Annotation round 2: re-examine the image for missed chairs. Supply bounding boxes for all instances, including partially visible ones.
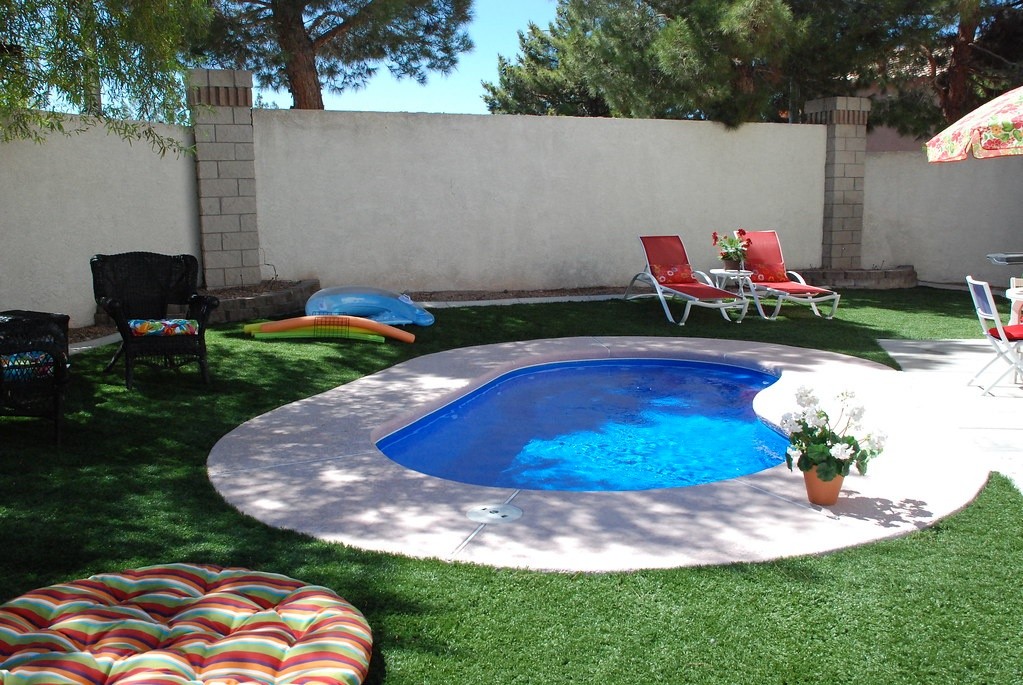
[88,251,219,392]
[732,229,841,320]
[1007,276,1023,384]
[965,273,1023,395]
[0,310,70,449]
[623,234,749,326]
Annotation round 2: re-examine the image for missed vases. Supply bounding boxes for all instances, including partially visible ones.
[803,464,845,506]
[723,259,740,272]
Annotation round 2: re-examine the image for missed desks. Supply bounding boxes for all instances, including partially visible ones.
[708,268,753,312]
[1004,286,1022,302]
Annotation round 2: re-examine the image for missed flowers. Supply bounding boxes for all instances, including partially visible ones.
[778,385,888,481]
[710,227,752,261]
